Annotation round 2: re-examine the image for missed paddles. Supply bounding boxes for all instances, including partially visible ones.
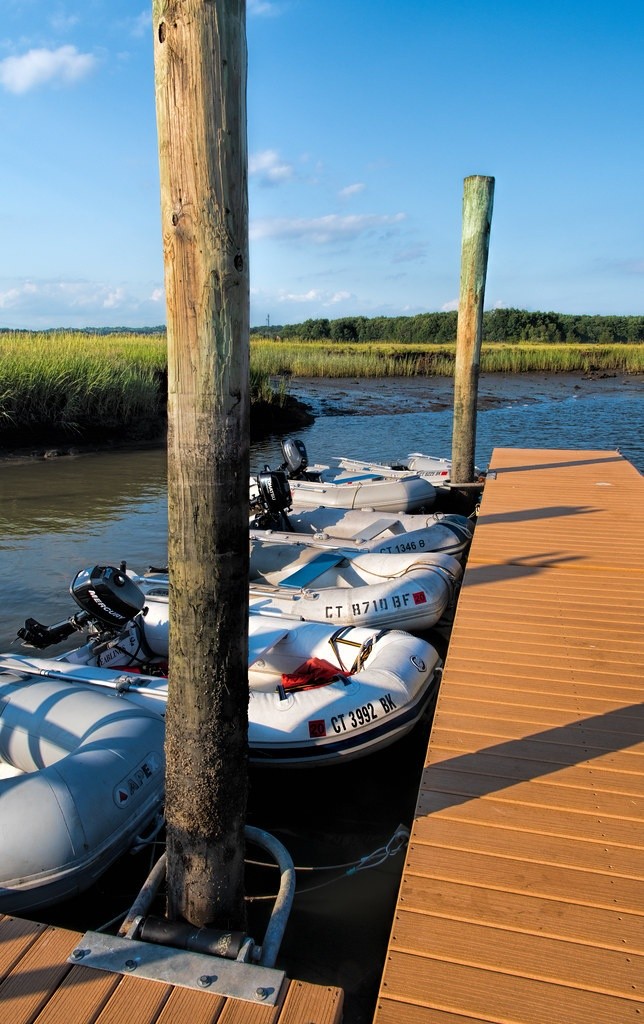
[250,476,325,493]
[0,656,168,697]
[143,593,303,622]
[118,568,295,600]
[406,452,478,470]
[331,456,391,470]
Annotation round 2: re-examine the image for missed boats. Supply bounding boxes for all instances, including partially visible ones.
[247,464,476,563]
[0,665,168,914]
[269,436,437,514]
[6,563,442,773]
[114,534,464,633]
[331,450,486,496]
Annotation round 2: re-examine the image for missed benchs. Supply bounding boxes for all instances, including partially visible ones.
[352,519,398,541]
[249,626,289,669]
[326,474,381,484]
[278,552,344,588]
